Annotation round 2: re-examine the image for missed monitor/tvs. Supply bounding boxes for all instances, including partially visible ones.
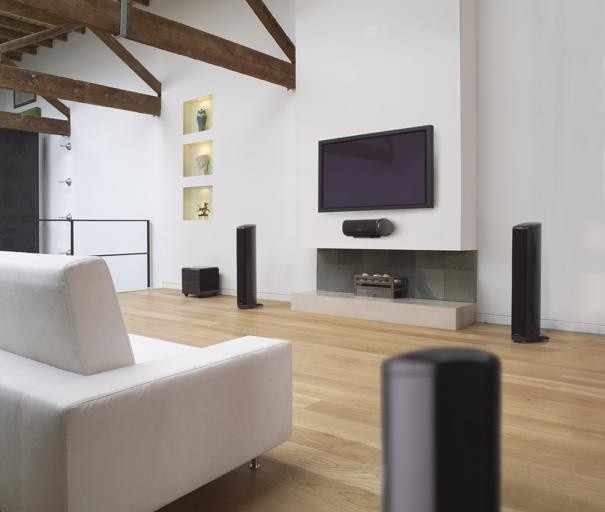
[317,125,436,211]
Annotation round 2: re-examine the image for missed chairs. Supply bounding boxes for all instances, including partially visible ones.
[2,249,294,512]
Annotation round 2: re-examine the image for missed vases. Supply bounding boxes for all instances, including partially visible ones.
[196,111,206,132]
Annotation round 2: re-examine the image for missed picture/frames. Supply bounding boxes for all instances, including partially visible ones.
[13,88,36,108]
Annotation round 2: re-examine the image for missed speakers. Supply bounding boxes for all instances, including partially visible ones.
[511,222,548,343]
[341,219,395,238]
[236,224,263,308]
